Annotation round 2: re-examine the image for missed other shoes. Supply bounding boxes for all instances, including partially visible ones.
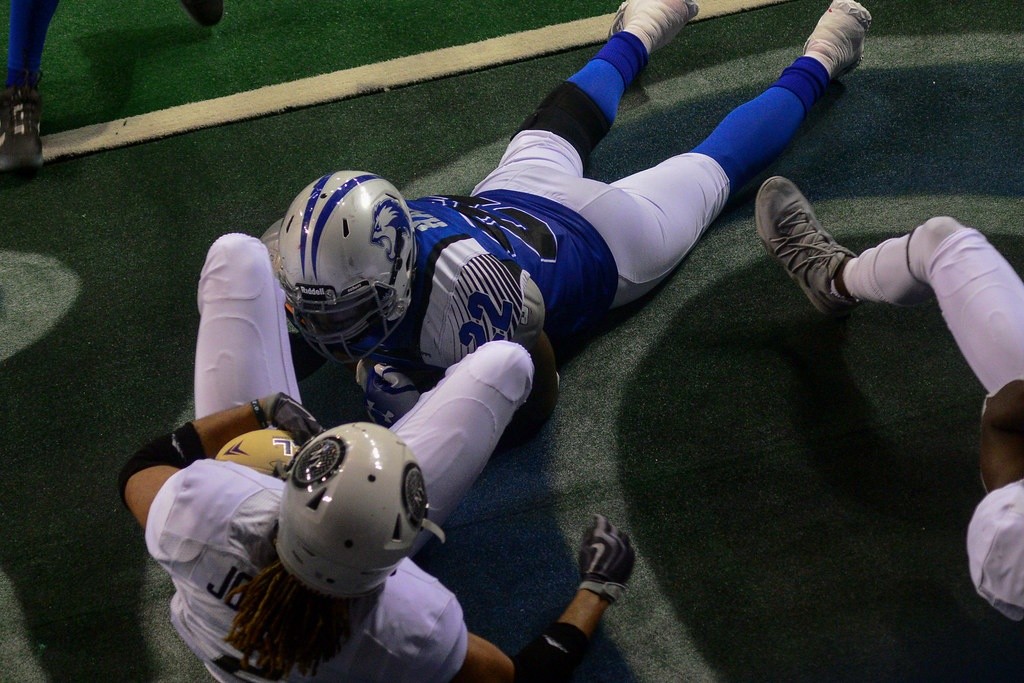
[607,0,699,40]
[0,70,44,171]
[804,0,872,74]
[181,0,224,25]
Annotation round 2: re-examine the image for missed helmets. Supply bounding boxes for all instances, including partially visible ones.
[278,170,417,362]
[275,422,430,596]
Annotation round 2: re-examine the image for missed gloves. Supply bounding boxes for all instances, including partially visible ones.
[266,391,324,447]
[364,362,420,429]
[579,514,635,604]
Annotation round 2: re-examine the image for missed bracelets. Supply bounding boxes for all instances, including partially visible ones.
[251,399,268,429]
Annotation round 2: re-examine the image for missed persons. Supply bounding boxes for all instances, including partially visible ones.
[118,231,637,683]
[0,0,223,174]
[256,0,874,427]
[755,176,1024,623]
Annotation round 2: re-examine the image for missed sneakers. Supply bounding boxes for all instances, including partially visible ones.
[754,176,863,319]
